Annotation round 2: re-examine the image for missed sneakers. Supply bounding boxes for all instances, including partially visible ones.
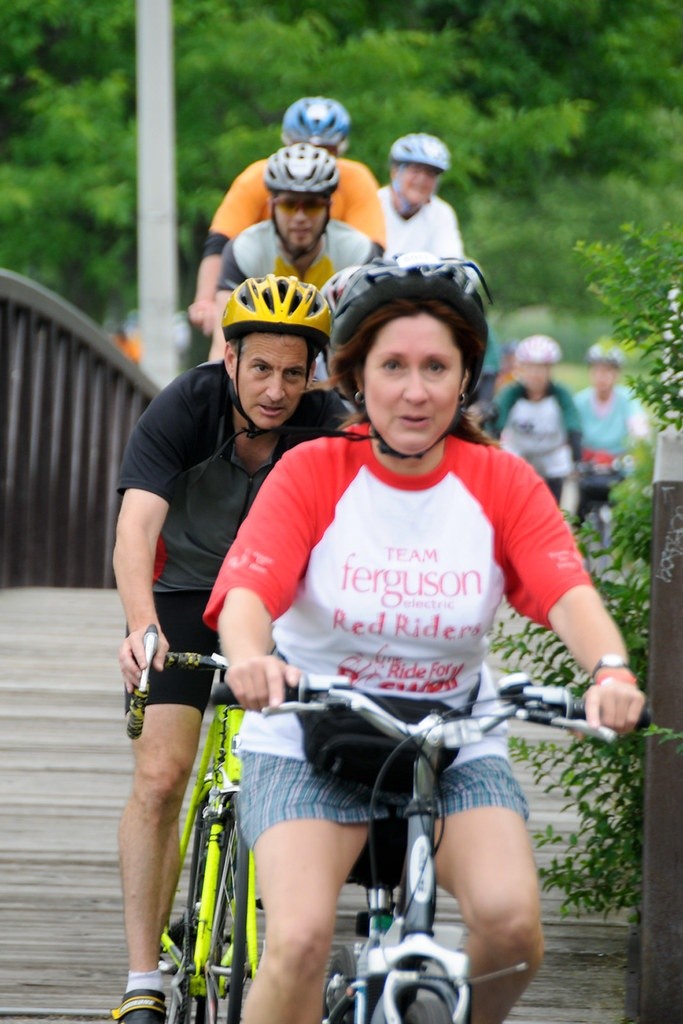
[110,988,169,1024]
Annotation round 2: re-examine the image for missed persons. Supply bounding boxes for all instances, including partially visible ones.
[185,98,385,335]
[486,336,584,516]
[112,276,356,1024]
[570,340,647,528]
[211,144,390,385]
[374,132,468,271]
[204,256,646,1024]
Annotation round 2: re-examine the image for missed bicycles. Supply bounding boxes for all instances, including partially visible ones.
[575,457,638,551]
[210,672,654,1021]
[128,627,268,1024]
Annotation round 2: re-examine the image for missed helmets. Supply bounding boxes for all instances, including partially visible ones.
[261,142,341,200]
[514,334,564,366]
[220,274,333,361]
[388,131,452,175]
[319,250,496,400]
[582,341,627,370]
[280,95,352,148]
[499,338,521,355]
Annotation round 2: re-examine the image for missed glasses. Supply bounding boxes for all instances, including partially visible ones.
[273,197,328,218]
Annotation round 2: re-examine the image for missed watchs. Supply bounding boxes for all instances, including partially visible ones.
[591,654,633,677]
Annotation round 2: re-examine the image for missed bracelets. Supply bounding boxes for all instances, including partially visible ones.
[596,671,638,686]
